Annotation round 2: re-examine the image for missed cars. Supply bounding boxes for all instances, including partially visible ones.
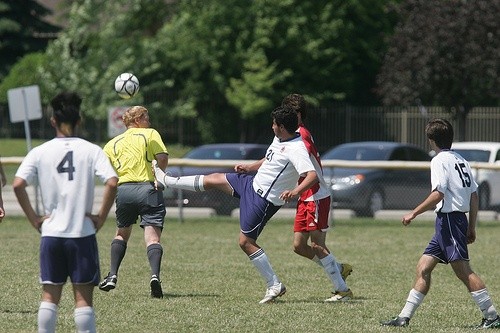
[163,143,269,216]
[320,141,432,217]
[451,141,500,210]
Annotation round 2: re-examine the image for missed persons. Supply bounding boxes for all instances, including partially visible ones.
[282,94,354,303]
[99,106,169,299]
[380,118,500,329]
[151,107,319,303]
[0,160,6,223]
[13,92,120,332]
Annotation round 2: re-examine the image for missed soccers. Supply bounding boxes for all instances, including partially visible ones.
[115,72,139,99]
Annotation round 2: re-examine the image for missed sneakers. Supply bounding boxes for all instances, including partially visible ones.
[151,160,168,192]
[149,275,163,299]
[473,315,500,330]
[335,264,353,295]
[259,282,286,304]
[379,316,410,328]
[325,289,353,303]
[99,272,117,292]
